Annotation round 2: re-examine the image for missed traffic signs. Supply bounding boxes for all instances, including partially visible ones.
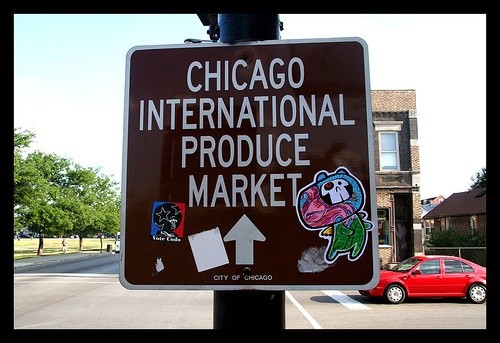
[120,35,380,292]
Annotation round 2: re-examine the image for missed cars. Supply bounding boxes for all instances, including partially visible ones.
[359,255,486,304]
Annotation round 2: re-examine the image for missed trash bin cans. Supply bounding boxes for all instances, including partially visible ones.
[107,244,112,252]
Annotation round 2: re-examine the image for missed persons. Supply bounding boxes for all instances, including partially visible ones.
[62,238,67,255]
